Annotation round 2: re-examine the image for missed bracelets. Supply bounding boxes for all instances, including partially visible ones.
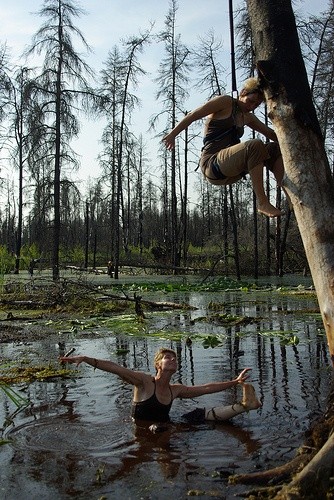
[93,358,98,374]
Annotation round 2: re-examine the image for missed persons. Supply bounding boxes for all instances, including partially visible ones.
[56,346,261,434]
[161,76,294,218]
